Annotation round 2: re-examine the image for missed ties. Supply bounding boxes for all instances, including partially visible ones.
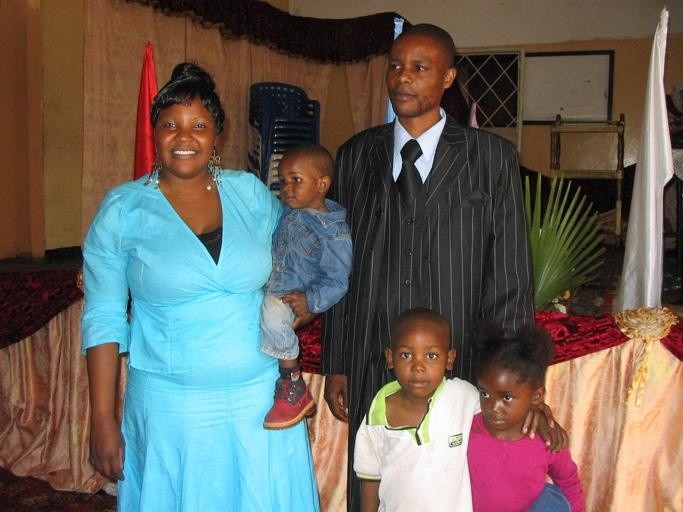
[395,139,423,210]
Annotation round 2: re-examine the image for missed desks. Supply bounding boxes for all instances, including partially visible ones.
[0,244,683,512]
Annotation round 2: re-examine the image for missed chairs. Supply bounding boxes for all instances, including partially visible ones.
[246,81,321,200]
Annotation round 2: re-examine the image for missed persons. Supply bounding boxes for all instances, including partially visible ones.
[322,23,553,512]
[352,307,570,512]
[466,316,586,511]
[79,63,320,512]
[258,144,353,430]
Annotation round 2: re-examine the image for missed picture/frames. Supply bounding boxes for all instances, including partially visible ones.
[515,50,614,125]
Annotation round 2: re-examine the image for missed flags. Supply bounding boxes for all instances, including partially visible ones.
[613,5,673,313]
[132,41,160,180]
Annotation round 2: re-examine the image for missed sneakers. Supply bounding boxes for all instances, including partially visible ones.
[263,375,317,429]
